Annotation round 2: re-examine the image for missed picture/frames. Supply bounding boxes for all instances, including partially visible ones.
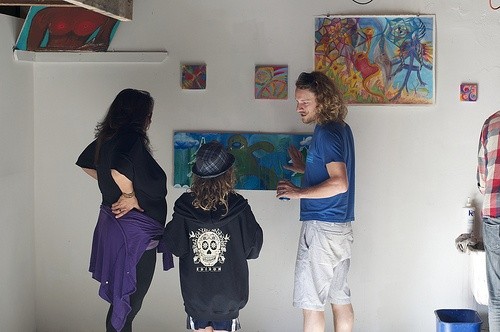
[311,12,438,108]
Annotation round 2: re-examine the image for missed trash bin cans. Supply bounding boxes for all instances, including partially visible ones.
[434,308,484,332]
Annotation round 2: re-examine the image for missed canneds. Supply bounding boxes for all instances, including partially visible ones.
[279,178,290,201]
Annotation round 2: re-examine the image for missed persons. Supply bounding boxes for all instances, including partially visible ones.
[74,89,168,332]
[275,71,355,332]
[163,143,264,332]
[476,110,500,332]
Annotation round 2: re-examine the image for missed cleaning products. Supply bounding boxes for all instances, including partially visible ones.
[461,197,476,234]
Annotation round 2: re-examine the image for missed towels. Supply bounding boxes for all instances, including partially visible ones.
[455,234,477,255]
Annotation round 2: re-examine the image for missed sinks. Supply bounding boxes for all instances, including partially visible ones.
[467,244,489,305]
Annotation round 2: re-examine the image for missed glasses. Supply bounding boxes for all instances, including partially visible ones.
[299,72,321,86]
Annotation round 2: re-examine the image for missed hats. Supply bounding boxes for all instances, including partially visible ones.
[192,142,235,179]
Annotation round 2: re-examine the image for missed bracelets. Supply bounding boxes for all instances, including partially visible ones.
[122,192,135,198]
